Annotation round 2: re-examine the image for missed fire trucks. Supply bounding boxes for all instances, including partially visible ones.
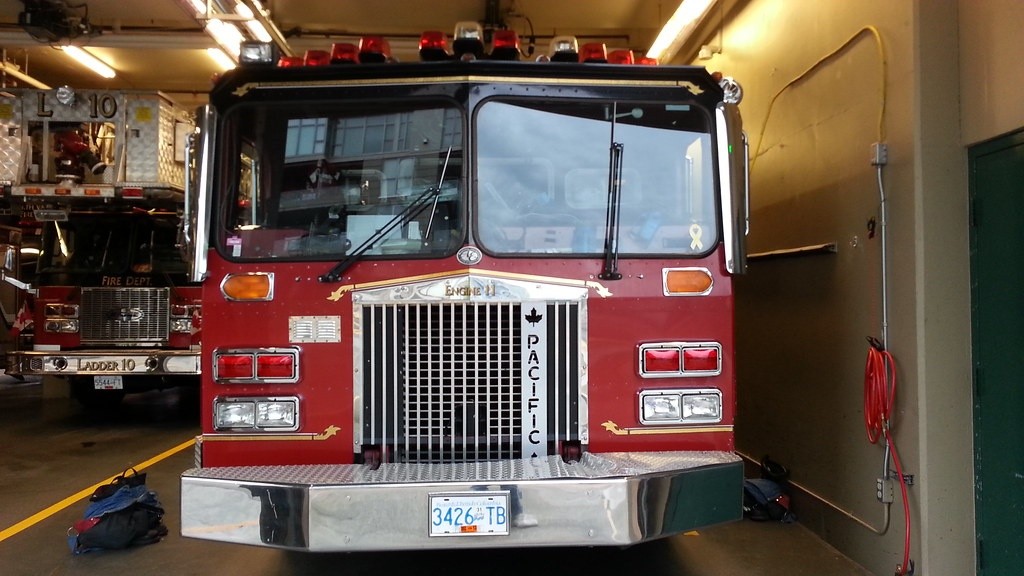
[179,24,748,553]
[1,82,251,403]
